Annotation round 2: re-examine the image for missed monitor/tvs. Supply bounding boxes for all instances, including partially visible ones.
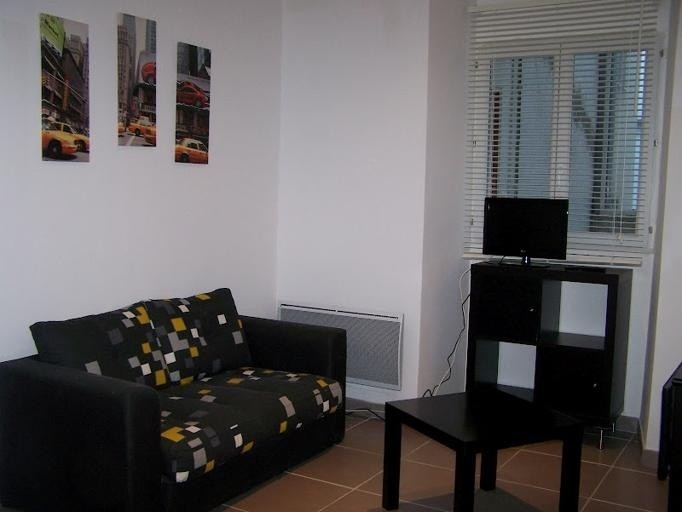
[482,197,569,269]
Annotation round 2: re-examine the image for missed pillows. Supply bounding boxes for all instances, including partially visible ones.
[29,299,171,390]
[149,287,253,386]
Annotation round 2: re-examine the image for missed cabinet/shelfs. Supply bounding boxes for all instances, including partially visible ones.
[658,360,682,512]
[465,261,633,449]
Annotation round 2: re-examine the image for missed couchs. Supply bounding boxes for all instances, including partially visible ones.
[0,315,347,512]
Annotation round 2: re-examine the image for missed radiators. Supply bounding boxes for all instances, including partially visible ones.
[278,302,403,391]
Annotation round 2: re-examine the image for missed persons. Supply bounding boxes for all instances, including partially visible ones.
[47,109,58,122]
